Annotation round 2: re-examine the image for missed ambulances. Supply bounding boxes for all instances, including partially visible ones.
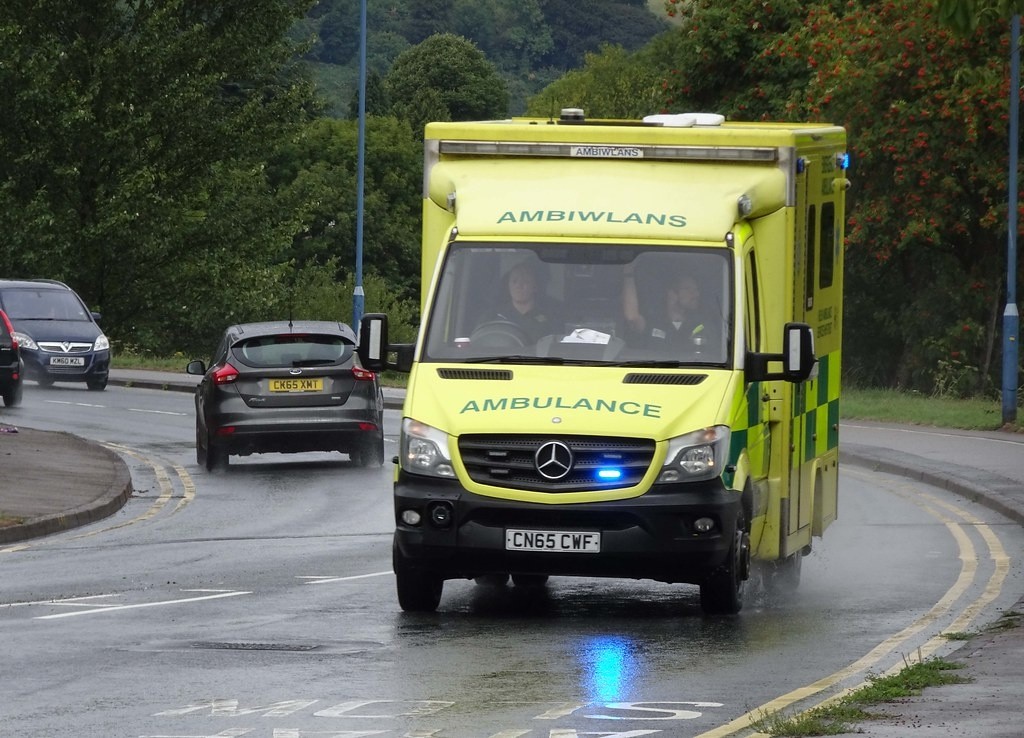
[359,107,851,613]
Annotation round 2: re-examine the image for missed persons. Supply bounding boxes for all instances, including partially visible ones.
[471,266,559,346]
[622,252,719,352]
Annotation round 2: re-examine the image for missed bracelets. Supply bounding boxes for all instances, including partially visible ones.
[624,273,634,277]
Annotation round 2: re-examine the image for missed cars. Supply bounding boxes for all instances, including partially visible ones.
[186,320,384,470]
[0,279,110,391]
